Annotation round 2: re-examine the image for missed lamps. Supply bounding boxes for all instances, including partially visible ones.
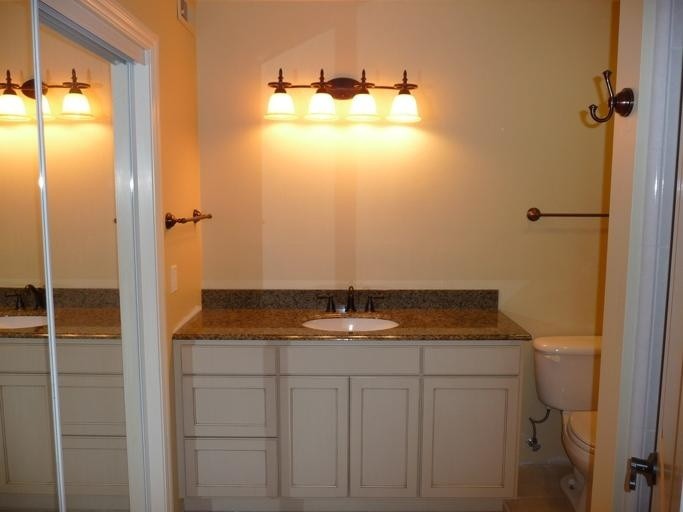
[0,69,93,114]
[262,68,423,122]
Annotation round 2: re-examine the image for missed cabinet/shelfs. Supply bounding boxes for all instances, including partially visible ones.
[419,339,518,500]
[277,340,419,502]
[172,339,277,502]
[0,338,57,496]
[51,336,128,499]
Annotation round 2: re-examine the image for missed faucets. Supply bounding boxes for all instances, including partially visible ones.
[346,285,357,313]
[23,284,45,311]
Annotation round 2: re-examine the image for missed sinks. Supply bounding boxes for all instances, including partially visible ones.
[297,314,400,332]
[0,312,59,331]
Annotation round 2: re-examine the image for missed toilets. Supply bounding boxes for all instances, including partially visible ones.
[531,333,602,512]
